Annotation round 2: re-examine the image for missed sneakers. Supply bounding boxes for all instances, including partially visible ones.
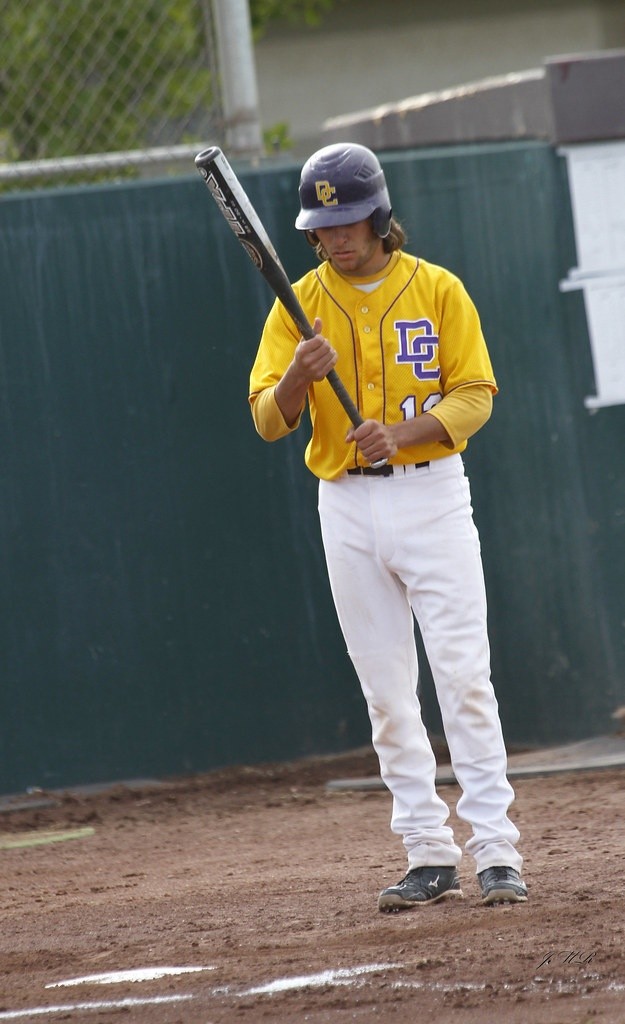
[477,867,527,905]
[378,866,463,913]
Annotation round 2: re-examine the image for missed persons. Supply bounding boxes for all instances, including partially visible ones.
[248,143,527,910]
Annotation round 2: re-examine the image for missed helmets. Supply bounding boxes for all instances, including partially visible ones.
[295,143,392,247]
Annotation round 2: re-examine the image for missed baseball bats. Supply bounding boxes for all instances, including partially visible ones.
[194,145,388,470]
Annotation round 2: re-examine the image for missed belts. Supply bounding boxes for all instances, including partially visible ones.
[346,460,429,477]
